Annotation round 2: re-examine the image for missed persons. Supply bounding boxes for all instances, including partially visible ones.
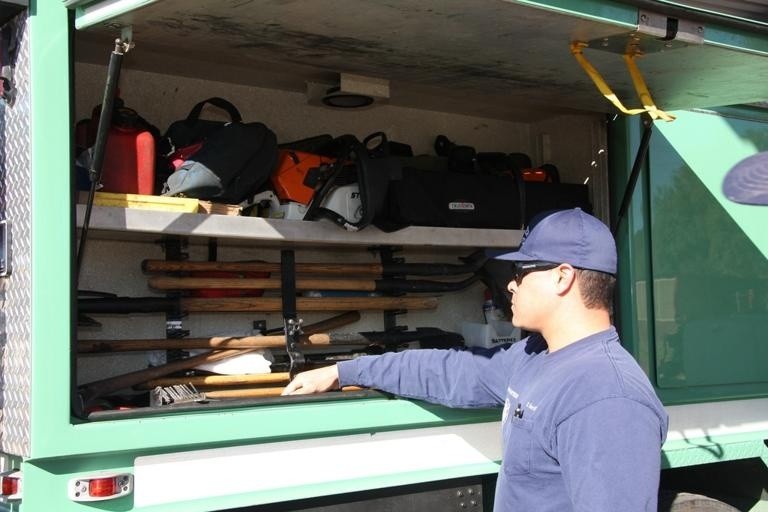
[281,208,670,512]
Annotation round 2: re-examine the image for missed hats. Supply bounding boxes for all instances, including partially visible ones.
[487,205,618,274]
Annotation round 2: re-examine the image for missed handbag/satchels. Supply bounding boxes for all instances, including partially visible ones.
[334,131,412,164]
[163,96,278,204]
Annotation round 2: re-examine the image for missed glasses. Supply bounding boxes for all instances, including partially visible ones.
[514,263,557,288]
